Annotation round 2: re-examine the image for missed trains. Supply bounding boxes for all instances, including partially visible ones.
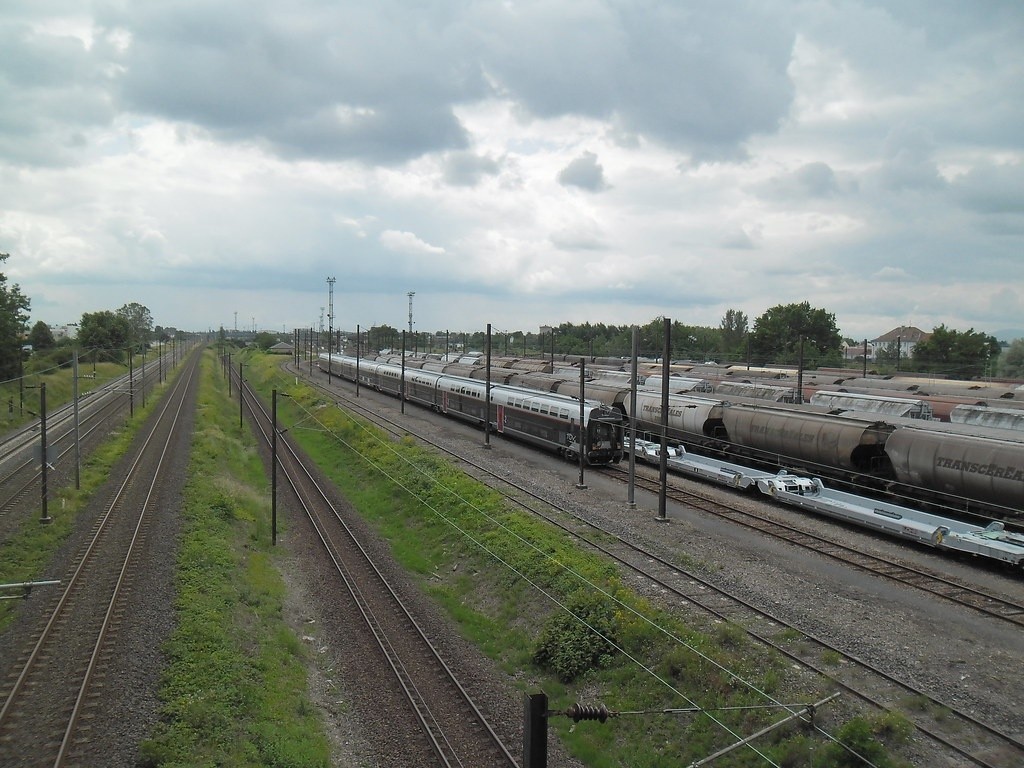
[318,353,624,468]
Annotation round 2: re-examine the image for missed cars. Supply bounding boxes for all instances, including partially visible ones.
[23,345,33,351]
